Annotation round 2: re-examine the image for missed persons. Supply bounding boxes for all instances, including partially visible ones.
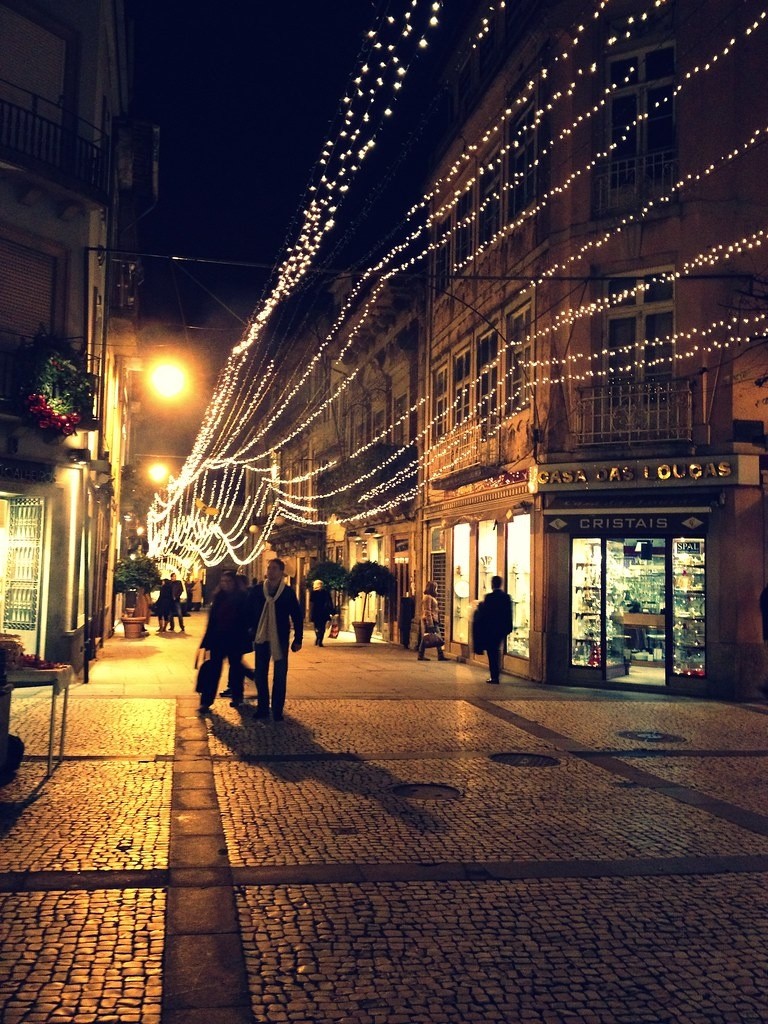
[477,576,512,684]
[196,558,303,720]
[309,579,336,647]
[154,573,185,632]
[417,581,449,661]
[180,578,202,616]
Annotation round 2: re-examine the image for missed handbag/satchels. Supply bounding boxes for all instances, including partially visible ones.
[195,660,209,693]
[328,618,339,639]
[423,628,445,647]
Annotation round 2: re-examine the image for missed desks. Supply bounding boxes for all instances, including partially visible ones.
[6,665,72,777]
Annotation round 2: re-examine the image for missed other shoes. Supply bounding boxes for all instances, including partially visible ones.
[181,627,185,631]
[317,641,323,647]
[195,707,207,716]
[418,657,430,661]
[251,709,268,719]
[316,640,318,645]
[168,628,174,631]
[249,669,257,681]
[273,710,283,721]
[438,656,450,661]
[219,689,231,697]
[230,699,241,706]
[486,678,500,684]
[157,628,166,632]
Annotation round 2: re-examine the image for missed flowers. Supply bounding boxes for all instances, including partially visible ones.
[30,354,94,438]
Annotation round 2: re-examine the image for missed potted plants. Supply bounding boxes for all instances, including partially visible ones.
[342,560,394,643]
[112,554,162,639]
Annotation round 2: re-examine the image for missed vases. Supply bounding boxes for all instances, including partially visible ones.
[126,607,135,618]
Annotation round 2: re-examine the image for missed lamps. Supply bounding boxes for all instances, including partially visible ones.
[346,532,360,538]
[247,511,260,534]
[513,500,534,512]
[275,513,287,527]
[365,528,378,535]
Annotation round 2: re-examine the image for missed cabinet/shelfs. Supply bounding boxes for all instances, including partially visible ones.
[575,562,706,650]
